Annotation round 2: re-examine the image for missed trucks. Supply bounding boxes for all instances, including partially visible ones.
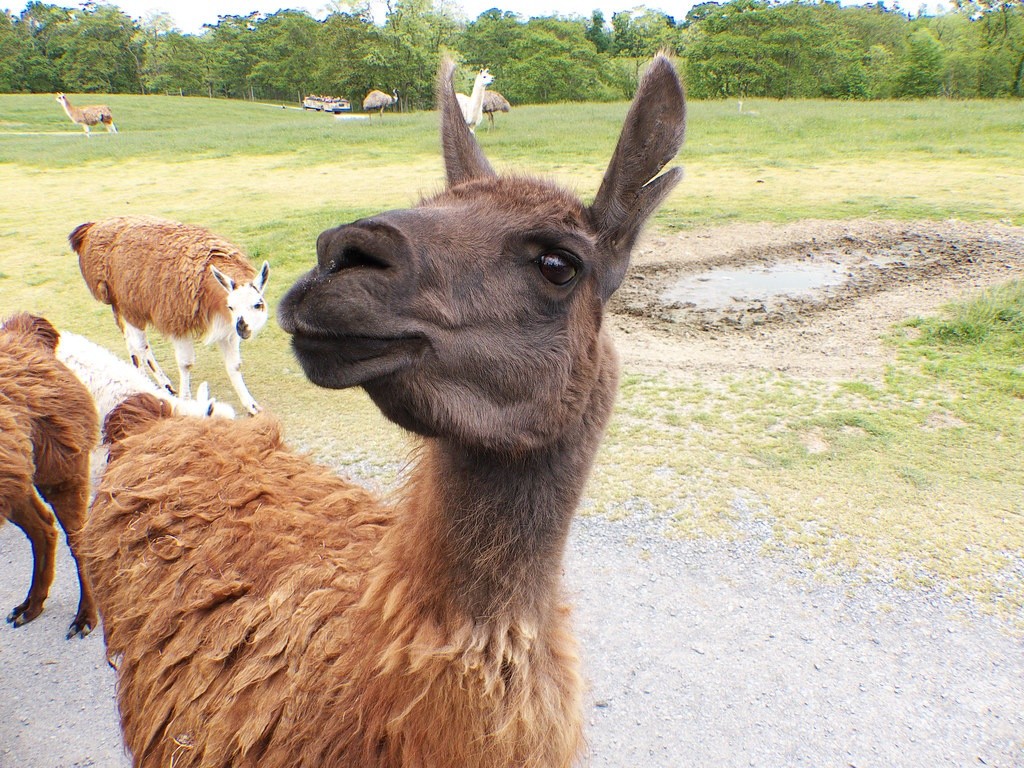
[302,94,352,114]
